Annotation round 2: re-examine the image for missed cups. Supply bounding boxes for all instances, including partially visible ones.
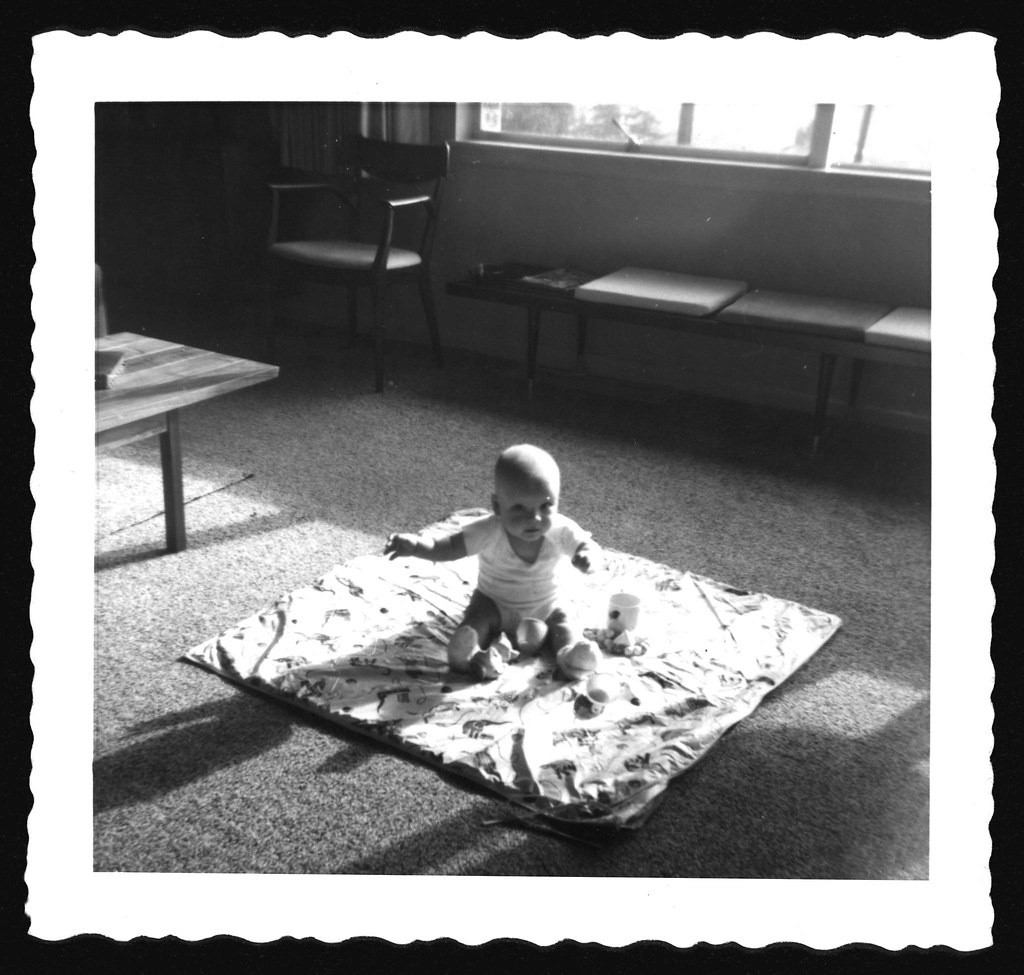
[608,593,639,634]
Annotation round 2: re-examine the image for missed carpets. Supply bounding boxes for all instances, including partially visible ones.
[187,506,844,827]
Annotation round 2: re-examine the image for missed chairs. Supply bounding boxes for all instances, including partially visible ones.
[257,135,448,394]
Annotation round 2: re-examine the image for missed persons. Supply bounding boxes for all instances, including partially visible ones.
[384,444,604,681]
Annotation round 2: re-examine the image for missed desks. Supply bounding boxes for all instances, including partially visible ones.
[95,332,279,557]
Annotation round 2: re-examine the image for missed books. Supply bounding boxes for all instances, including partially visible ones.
[95,350,125,389]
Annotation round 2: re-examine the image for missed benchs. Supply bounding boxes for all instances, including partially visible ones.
[443,261,930,457]
